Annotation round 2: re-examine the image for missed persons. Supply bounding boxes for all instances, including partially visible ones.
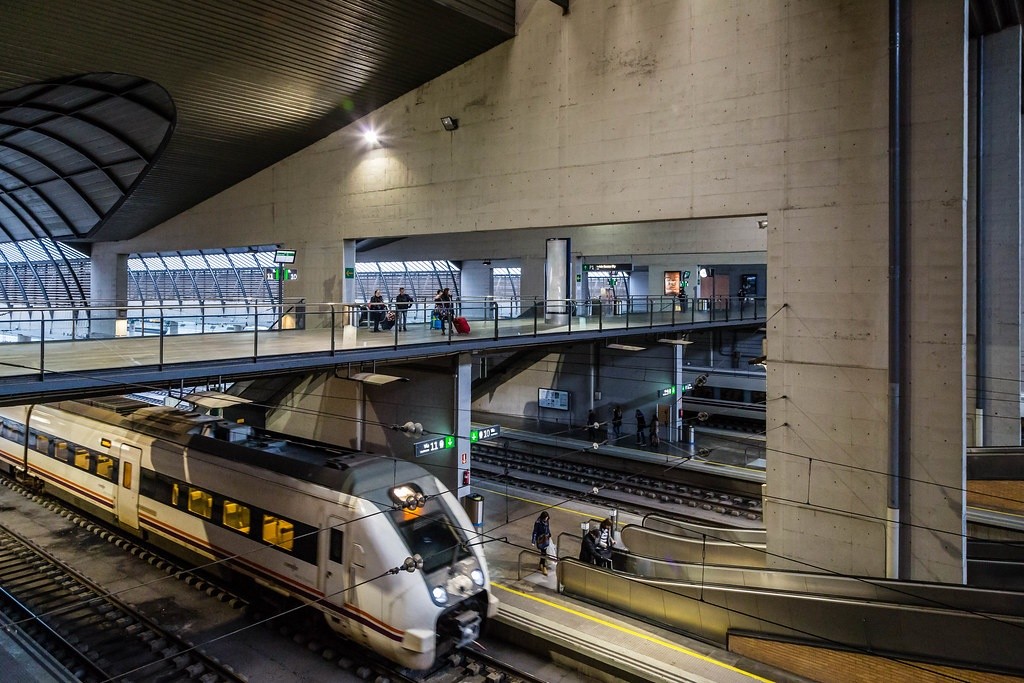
[635,409,647,446]
[394,287,414,331]
[645,413,659,447]
[611,405,622,439]
[530,511,551,576]
[586,409,596,442]
[432,287,456,336]
[577,529,607,565]
[369,289,386,332]
[678,289,688,313]
[593,517,615,566]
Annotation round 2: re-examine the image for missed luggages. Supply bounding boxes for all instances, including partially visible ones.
[433,318,441,329]
[452,314,470,334]
[382,317,395,330]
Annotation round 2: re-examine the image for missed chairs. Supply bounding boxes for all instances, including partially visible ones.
[225,503,242,529]
[75,450,88,469]
[242,507,250,527]
[264,517,279,545]
[97,457,109,476]
[190,491,204,515]
[57,442,68,459]
[206,498,212,517]
[280,525,293,550]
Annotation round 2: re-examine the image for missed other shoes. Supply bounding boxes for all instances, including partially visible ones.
[636,442,641,444]
[539,564,543,571]
[403,327,407,331]
[641,443,646,447]
[544,567,548,574]
[374,329,381,332]
[399,328,401,331]
[441,332,446,335]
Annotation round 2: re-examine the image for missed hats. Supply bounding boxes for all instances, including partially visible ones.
[592,529,602,538]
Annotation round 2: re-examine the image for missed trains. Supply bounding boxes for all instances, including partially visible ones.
[682,367,766,428]
[1,395,500,674]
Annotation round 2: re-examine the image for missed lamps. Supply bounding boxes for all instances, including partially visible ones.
[440,116,458,131]
[483,259,491,265]
[758,220,768,229]
[700,268,714,278]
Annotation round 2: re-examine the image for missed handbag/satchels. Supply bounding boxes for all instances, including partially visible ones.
[547,538,557,558]
[596,546,611,558]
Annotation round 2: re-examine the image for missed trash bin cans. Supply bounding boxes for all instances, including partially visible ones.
[282,298,306,330]
[466,493,484,524]
[682,424,695,444]
[699,299,708,311]
